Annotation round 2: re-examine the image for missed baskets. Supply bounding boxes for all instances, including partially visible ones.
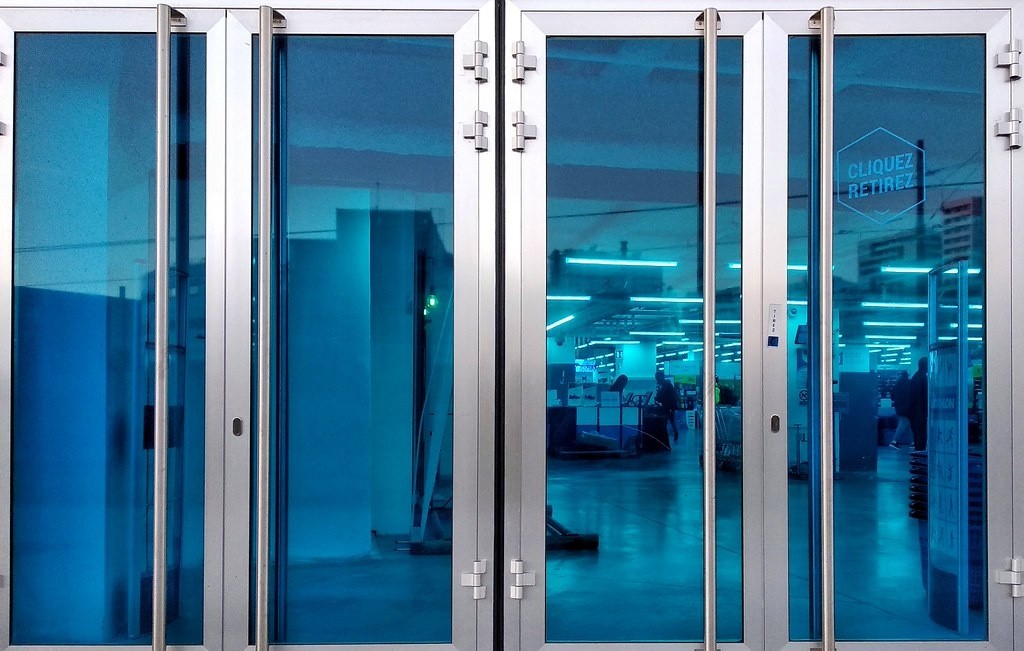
[909,441,987,609]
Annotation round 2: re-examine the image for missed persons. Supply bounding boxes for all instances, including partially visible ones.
[656,373,679,441]
[609,375,628,400]
[889,370,912,450]
[716,375,721,406]
[907,358,929,450]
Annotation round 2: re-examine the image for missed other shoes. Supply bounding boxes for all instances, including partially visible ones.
[674,432,678,441]
[889,441,900,449]
